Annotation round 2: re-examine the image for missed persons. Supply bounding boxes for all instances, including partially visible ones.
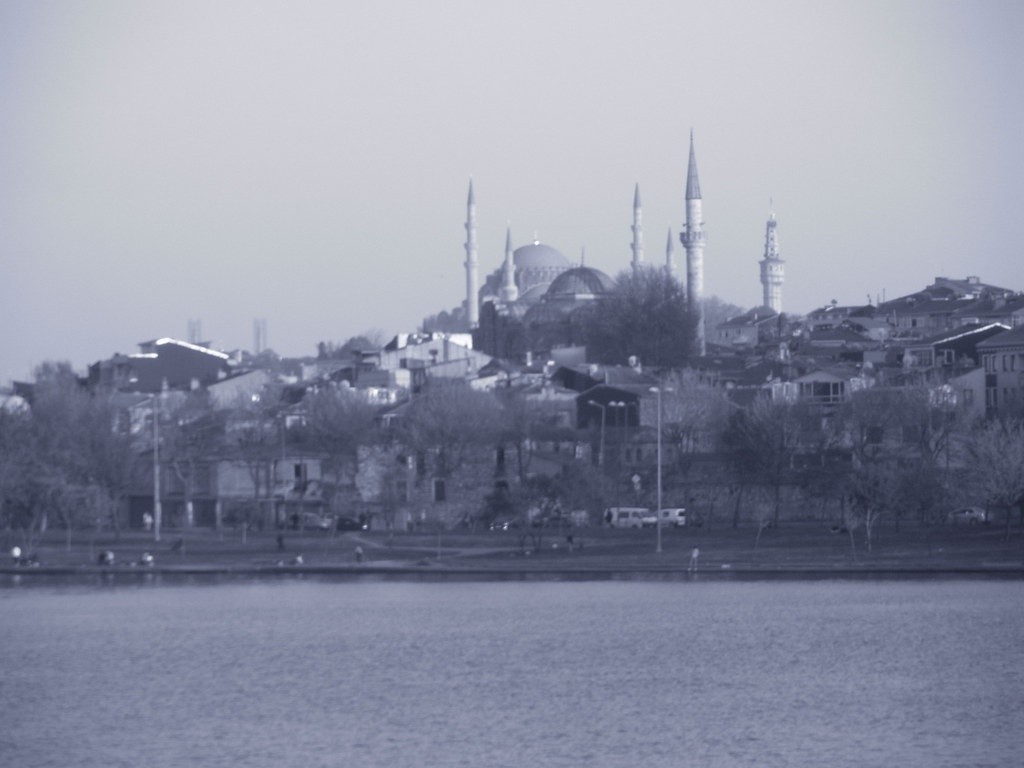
[687,541,701,571]
[276,532,286,554]
[566,532,573,552]
[354,542,364,563]
[12,545,21,564]
[142,512,153,532]
[290,512,299,529]
[169,537,185,554]
[359,511,366,530]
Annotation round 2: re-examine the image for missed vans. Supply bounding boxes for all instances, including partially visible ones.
[653,507,686,527]
[603,506,657,530]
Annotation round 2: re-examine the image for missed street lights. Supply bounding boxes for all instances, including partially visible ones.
[587,399,605,464]
[608,400,625,442]
[648,377,675,552]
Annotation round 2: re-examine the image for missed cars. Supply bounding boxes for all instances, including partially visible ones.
[944,507,994,525]
[279,510,369,530]
